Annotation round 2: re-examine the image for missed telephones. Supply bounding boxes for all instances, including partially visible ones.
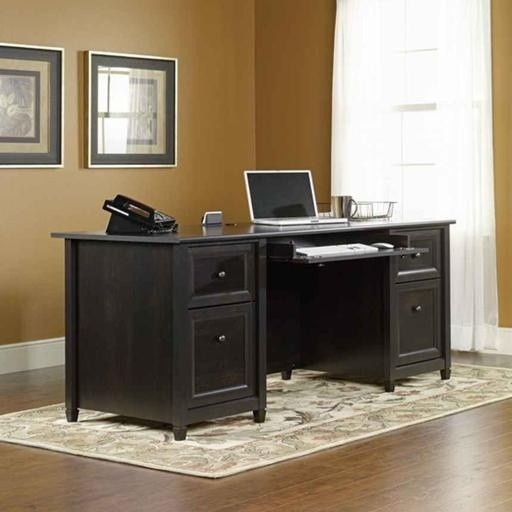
[103,194,176,227]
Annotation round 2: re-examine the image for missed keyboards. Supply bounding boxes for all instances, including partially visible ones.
[295,243,379,258]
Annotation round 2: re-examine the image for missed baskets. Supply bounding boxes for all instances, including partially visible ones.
[347,202,397,219]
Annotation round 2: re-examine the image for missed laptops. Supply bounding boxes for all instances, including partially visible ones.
[244,169,348,226]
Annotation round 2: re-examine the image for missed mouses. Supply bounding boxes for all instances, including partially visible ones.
[371,241,394,249]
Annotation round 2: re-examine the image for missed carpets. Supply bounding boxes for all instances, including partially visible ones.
[1,362,512,480]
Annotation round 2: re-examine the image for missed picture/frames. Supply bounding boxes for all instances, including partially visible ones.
[0,42,65,168]
[85,49,179,168]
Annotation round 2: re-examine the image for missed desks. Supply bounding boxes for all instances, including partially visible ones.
[43,219,458,441]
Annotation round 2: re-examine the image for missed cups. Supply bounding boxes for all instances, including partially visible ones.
[331,196,358,221]
